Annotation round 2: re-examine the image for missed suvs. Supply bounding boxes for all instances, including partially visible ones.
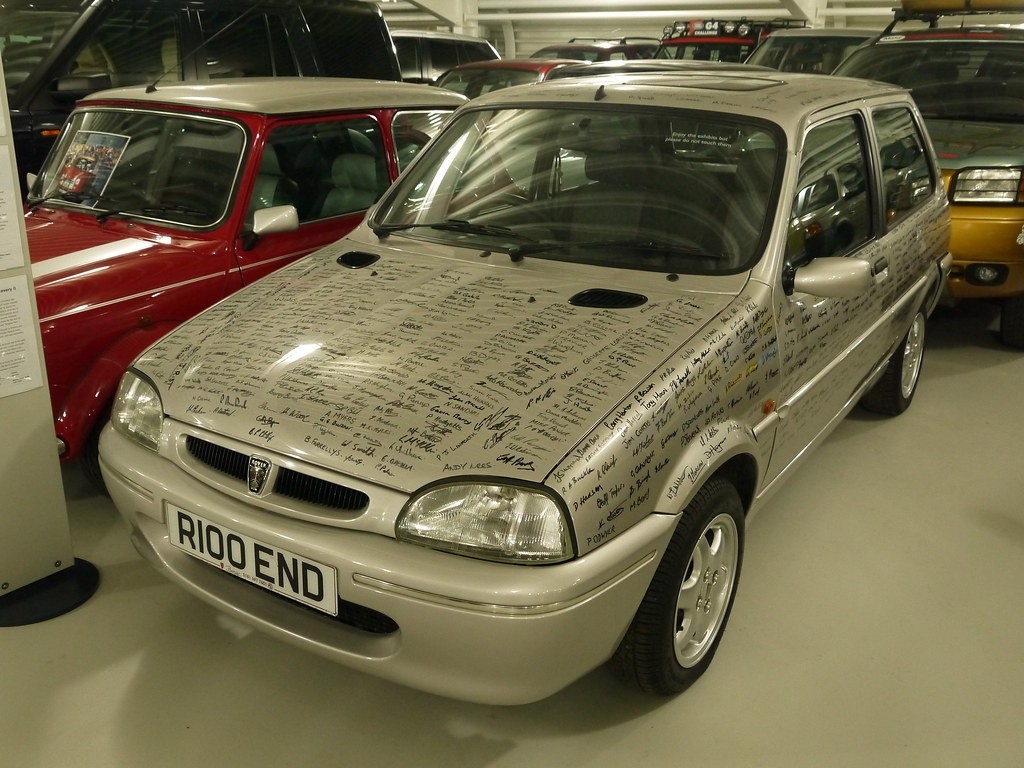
[434,0,1024,353]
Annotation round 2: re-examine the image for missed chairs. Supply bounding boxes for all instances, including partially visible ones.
[698,148,777,270]
[319,151,376,218]
[561,145,649,244]
[219,143,290,231]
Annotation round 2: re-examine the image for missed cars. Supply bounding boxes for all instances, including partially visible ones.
[24,0,527,494]
[96,69,952,707]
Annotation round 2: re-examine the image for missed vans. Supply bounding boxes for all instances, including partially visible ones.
[0,0,501,207]
